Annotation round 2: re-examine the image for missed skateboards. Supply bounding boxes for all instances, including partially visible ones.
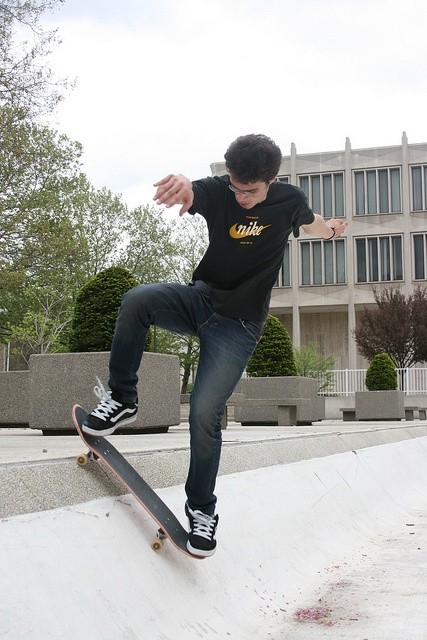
[72,403,206,559]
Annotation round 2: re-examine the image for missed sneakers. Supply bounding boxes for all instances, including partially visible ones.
[184,501,219,558]
[82,376,138,437]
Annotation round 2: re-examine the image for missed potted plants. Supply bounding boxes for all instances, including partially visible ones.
[233,312,326,425]
[356,352,406,420]
[29,266,180,433]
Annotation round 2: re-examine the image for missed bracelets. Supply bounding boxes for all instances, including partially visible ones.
[324,226,335,240]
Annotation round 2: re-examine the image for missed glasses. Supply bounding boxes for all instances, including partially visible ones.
[227,184,269,197]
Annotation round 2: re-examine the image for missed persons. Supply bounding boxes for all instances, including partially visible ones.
[80,133,352,553]
